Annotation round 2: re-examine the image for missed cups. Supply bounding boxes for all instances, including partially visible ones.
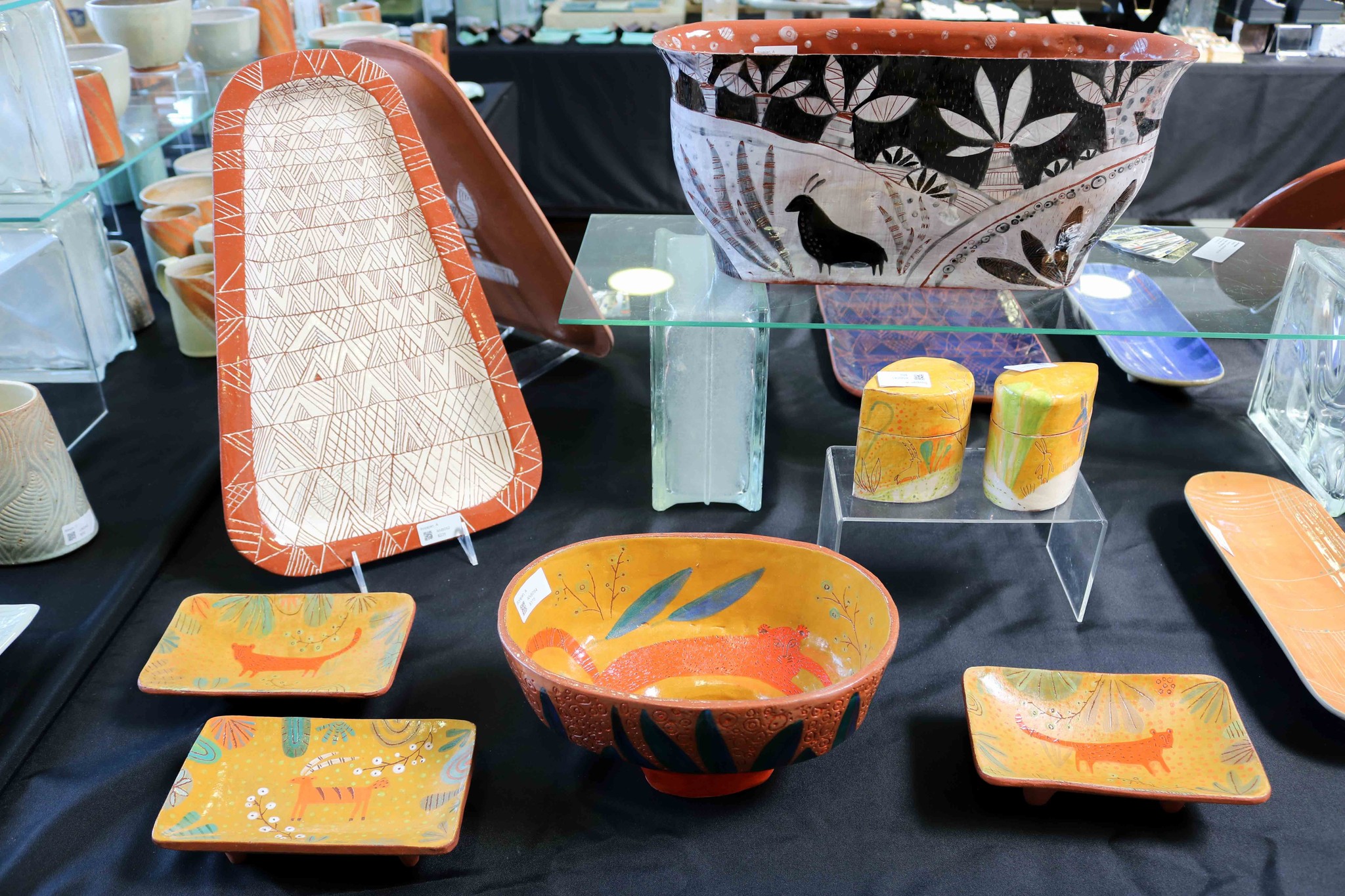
[71,68,125,168]
[140,204,216,340]
[107,240,155,332]
[336,0,381,23]
[410,21,449,76]
[0,378,99,564]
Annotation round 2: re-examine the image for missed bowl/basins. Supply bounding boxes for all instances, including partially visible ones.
[65,44,130,120]
[186,6,260,73]
[137,146,213,225]
[494,533,902,799]
[307,22,399,49]
[84,0,192,69]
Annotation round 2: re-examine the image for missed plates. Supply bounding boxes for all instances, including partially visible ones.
[149,714,478,866]
[1184,470,1345,723]
[135,591,418,698]
[1063,262,1226,388]
[815,284,1053,403]
[342,39,614,358]
[962,666,1273,813]
[212,63,544,578]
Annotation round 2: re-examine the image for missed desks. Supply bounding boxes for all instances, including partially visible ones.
[1,28,1345,896]
